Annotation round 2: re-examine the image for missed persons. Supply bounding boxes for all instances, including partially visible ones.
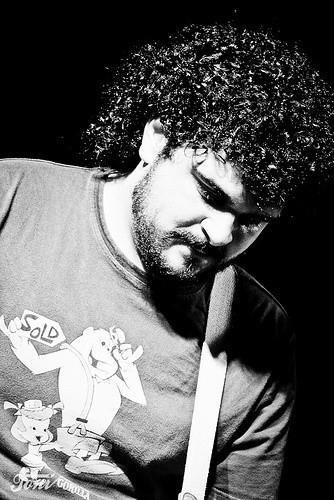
[0,22,334,500]
